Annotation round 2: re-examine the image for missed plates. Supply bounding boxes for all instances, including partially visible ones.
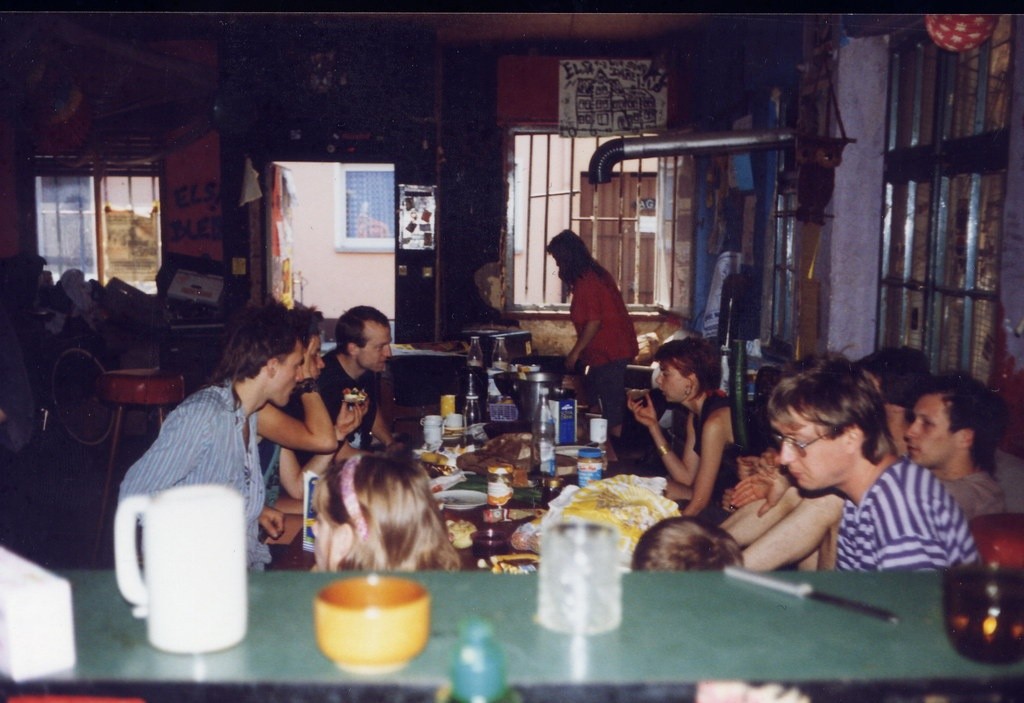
[556,445,592,457]
[433,490,488,511]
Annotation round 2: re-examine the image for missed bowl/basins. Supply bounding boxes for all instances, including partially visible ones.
[312,577,431,675]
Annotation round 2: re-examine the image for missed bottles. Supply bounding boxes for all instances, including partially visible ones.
[485,461,514,510]
[940,510,1024,666]
[491,336,509,371]
[464,388,481,427]
[530,388,556,478]
[576,448,603,489]
[466,335,483,368]
[531,373,550,427]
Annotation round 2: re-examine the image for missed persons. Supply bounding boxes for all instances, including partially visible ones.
[547,231,639,445]
[311,454,460,575]
[258,310,369,570]
[630,515,748,581]
[318,305,405,471]
[118,319,306,573]
[627,337,750,526]
[0,303,34,556]
[717,346,1010,573]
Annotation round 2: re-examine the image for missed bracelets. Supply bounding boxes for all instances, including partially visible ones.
[299,378,318,395]
[659,444,672,457]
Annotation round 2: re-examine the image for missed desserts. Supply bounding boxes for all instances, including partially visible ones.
[342,388,367,403]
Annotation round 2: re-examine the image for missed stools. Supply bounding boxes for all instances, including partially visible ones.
[84,370,187,563]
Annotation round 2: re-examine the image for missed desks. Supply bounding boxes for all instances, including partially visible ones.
[3,567,1024,702]
[406,413,742,568]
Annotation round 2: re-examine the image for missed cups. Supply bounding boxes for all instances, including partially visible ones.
[112,482,249,656]
[590,418,608,444]
[469,530,508,561]
[423,415,442,444]
[535,521,624,636]
[440,394,456,419]
[445,413,463,427]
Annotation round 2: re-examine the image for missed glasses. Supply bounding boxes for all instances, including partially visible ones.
[770,426,854,457]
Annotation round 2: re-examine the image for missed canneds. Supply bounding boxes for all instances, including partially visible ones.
[577,448,605,487]
[487,464,514,506]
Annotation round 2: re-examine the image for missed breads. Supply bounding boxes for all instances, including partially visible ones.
[456,432,534,486]
